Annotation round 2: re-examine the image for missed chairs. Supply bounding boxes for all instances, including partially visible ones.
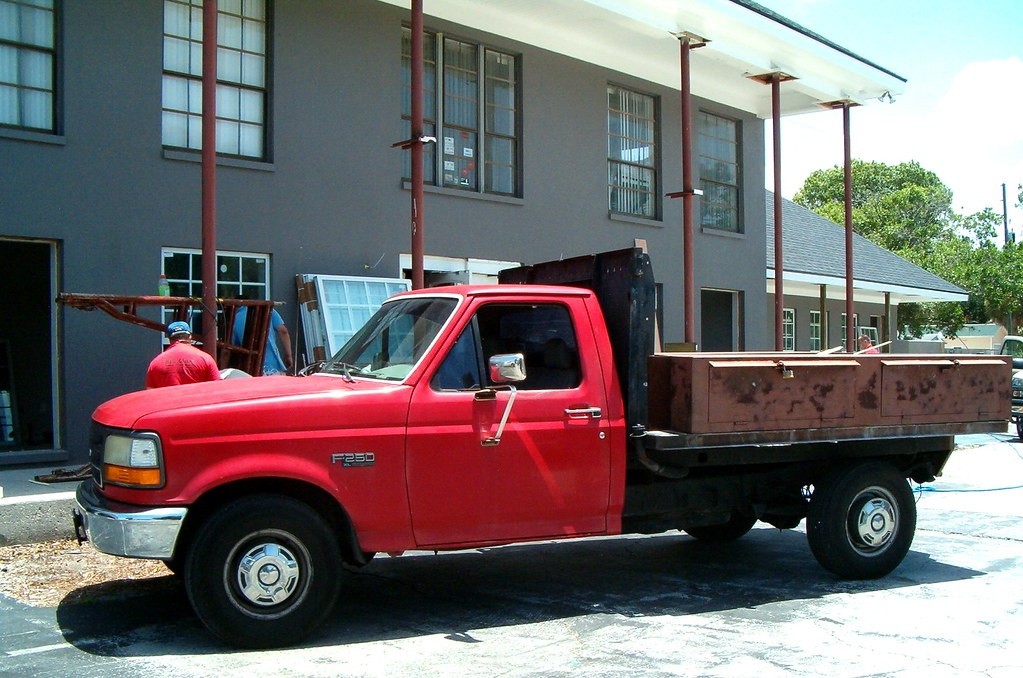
[543,338,579,390]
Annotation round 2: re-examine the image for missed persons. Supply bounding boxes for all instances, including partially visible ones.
[231,294,293,375]
[858,334,879,354]
[144,321,221,390]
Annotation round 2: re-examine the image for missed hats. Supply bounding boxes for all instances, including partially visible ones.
[168,321,192,337]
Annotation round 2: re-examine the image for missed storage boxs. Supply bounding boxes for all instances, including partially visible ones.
[647,351,1013,433]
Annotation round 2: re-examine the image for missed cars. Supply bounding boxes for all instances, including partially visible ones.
[996,335,1023,440]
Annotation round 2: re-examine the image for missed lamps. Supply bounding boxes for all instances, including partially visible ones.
[878,91,896,104]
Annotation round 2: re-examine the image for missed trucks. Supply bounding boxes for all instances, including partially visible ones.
[32,246,1014,644]
